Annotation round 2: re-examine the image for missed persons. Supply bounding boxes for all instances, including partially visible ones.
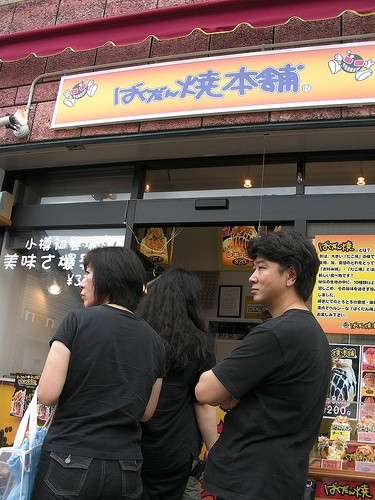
[132,266,218,500]
[194,228,332,500]
[30,246,167,500]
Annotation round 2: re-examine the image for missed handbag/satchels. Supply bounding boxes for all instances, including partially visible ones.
[0,386,59,500]
